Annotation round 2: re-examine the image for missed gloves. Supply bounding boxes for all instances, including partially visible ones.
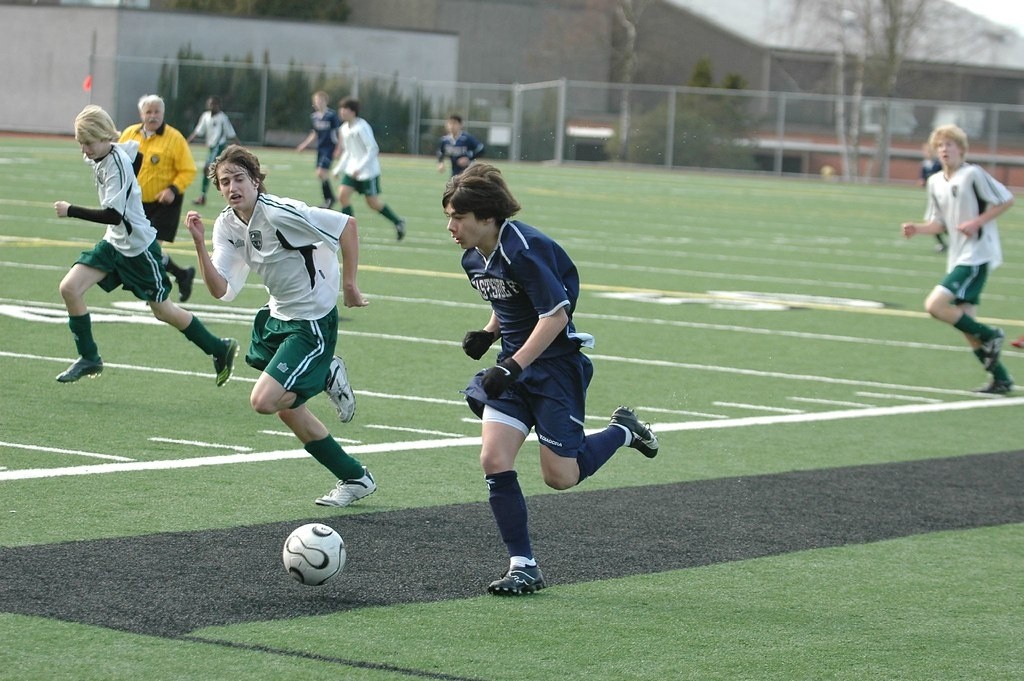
[462,329,494,360]
[482,357,522,401]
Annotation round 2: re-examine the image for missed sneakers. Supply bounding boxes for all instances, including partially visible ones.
[610,405,658,458]
[175,265,195,302]
[315,465,377,507]
[395,217,406,242]
[973,375,1015,394]
[488,562,546,596]
[982,327,1004,372]
[213,338,240,387]
[1011,335,1024,348]
[326,355,356,424]
[56,356,104,382]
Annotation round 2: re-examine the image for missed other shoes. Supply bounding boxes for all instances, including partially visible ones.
[192,199,205,205]
[325,196,336,209]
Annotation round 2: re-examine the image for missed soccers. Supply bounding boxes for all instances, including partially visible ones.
[282,522,347,586]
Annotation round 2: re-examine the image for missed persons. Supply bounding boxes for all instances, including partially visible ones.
[442,161,660,594]
[902,126,1014,394]
[183,145,378,507]
[117,95,196,302]
[54,104,241,388]
[332,97,404,241]
[436,115,485,175]
[295,92,342,209]
[917,141,950,252]
[186,96,241,205]
